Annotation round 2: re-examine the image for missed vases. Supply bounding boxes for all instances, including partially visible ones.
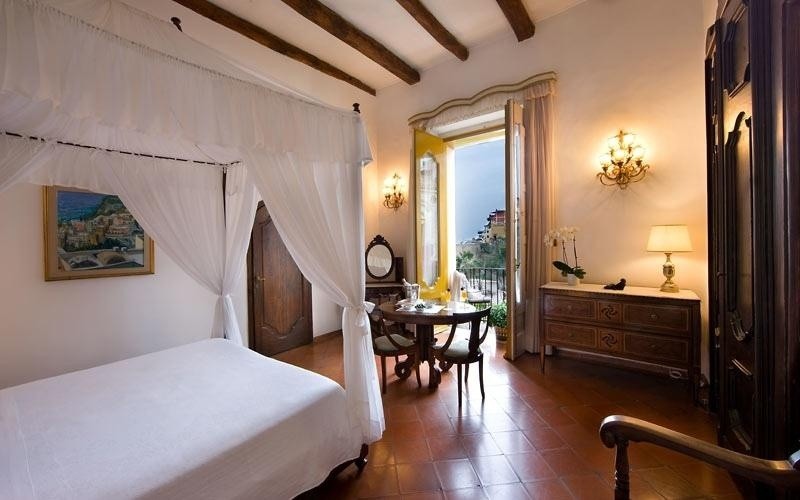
[567,275,580,285]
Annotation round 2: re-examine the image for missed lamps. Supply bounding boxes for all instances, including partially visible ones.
[383,174,405,211]
[647,225,693,293]
[596,130,650,188]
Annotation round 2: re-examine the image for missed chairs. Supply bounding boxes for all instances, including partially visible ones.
[366,309,422,393]
[427,306,494,410]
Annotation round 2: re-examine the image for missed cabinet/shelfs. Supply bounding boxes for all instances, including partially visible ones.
[705,0,800,500]
[538,282,701,403]
[364,283,405,334]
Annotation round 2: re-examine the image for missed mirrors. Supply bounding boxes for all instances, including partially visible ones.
[364,235,394,281]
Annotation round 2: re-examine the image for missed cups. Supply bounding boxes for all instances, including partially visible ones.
[414,307,424,311]
[425,303,434,308]
[401,303,413,309]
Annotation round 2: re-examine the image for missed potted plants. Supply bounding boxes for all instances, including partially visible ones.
[486,303,508,341]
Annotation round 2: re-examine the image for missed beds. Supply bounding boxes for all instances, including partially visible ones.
[1,339,385,500]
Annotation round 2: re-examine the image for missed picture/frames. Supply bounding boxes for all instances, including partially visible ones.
[43,187,154,281]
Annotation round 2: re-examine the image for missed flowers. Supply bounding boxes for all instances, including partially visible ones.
[543,227,586,278]
